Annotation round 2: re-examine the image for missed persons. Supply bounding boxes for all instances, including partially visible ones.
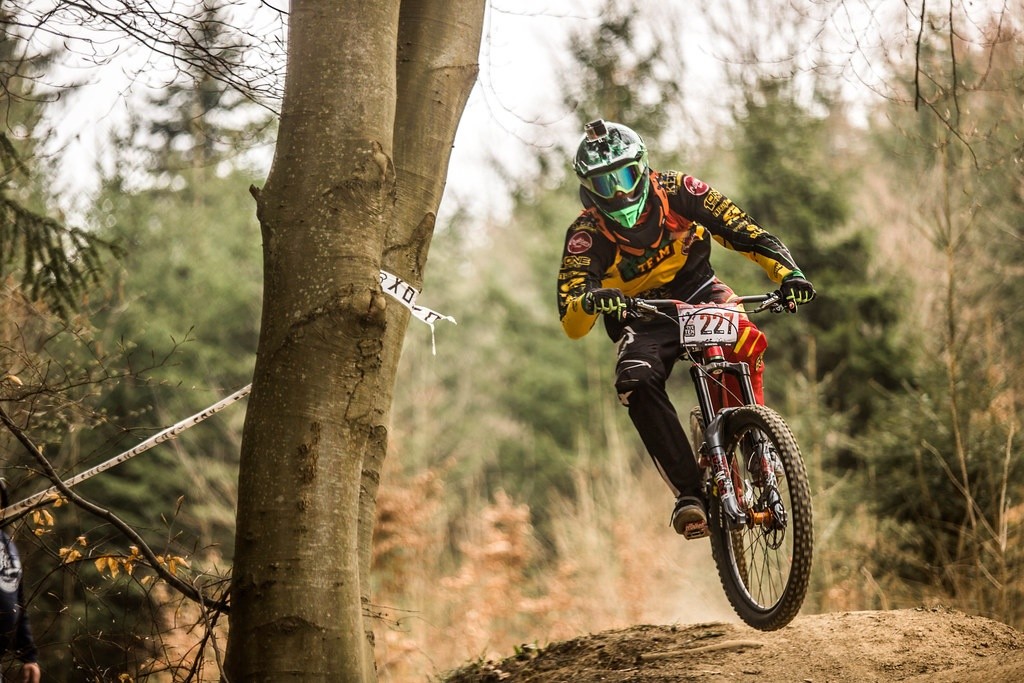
[556,116,817,541]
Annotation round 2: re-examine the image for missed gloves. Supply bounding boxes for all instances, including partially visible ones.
[779,276,817,314]
[586,286,627,323]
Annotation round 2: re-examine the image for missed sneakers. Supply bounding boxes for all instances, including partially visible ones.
[747,449,783,477]
[672,497,707,534]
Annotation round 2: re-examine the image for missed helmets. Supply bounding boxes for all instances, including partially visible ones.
[574,119,650,231]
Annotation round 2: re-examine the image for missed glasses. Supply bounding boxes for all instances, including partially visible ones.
[576,161,648,198]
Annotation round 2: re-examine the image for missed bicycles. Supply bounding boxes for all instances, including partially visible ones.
[600,288,815,632]
